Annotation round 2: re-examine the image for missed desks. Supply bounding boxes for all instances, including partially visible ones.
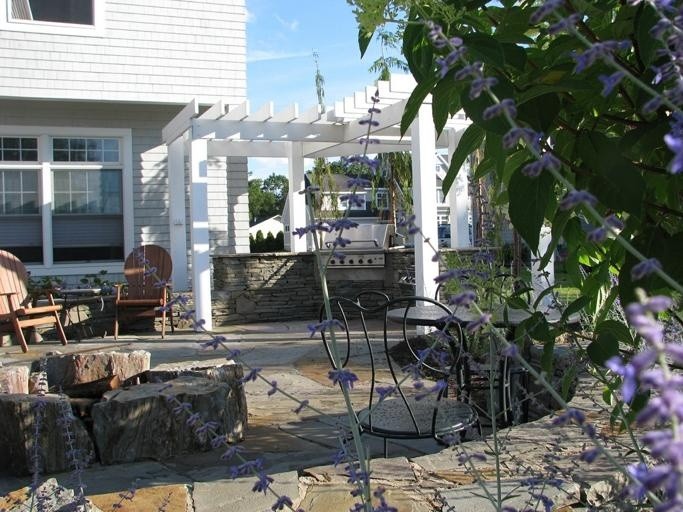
[57,289,106,342]
[387,307,581,428]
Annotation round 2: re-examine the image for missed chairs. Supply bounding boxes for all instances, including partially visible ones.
[436,275,531,426]
[319,290,479,458]
[113,245,175,339]
[0,249,68,354]
